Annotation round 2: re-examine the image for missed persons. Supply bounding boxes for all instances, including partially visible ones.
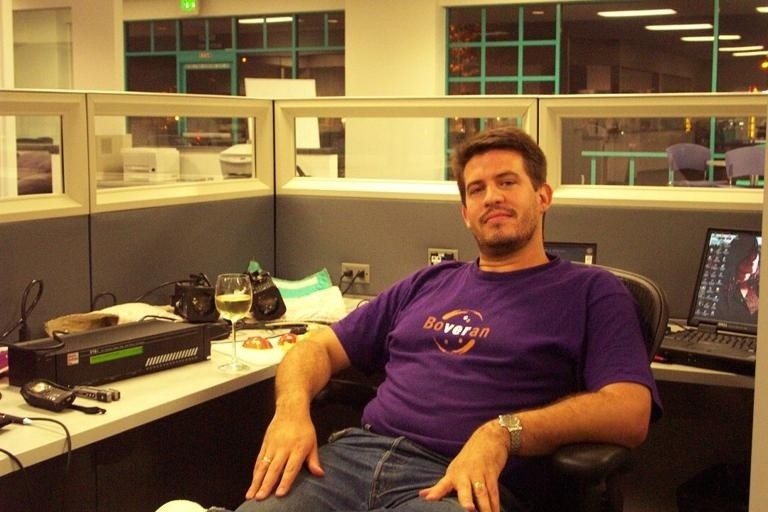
[233,127,665,512]
[715,234,760,326]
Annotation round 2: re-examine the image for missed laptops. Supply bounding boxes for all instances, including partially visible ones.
[660,227,763,365]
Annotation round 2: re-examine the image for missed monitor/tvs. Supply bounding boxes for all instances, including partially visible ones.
[544,239,599,265]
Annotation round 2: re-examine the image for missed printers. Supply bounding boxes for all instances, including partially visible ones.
[122,147,178,183]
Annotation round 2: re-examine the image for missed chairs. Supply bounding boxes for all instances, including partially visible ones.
[555,255,669,479]
[724,146,765,188]
[666,143,718,186]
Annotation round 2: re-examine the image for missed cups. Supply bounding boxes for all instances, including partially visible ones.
[214,273,253,374]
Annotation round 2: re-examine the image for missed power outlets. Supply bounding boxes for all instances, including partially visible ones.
[427,248,459,268]
[341,262,369,284]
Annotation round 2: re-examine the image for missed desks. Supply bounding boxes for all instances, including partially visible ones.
[1,294,368,479]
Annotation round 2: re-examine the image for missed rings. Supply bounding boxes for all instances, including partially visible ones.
[473,482,486,488]
[263,457,271,463]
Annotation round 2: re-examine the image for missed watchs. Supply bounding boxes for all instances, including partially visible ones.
[499,414,523,455]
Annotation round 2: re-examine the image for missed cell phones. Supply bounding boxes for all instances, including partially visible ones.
[21,378,76,412]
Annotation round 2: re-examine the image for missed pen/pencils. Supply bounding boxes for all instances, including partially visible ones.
[0,412,33,426]
